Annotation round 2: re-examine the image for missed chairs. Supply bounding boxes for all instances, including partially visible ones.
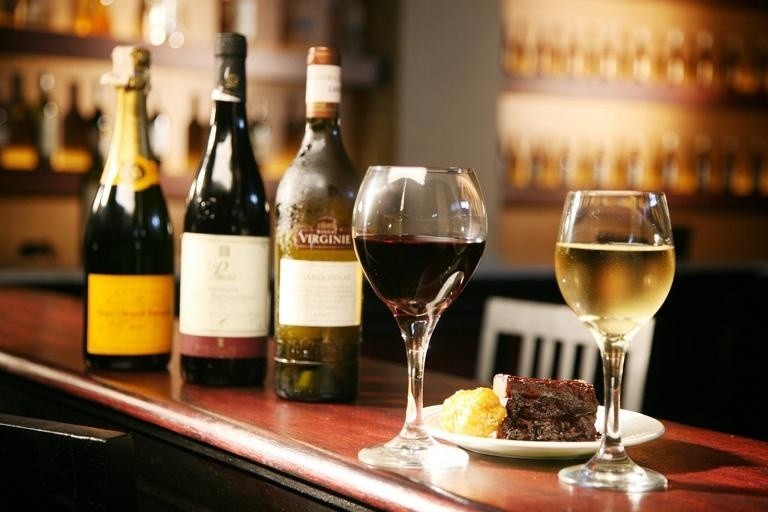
[477,297,655,412]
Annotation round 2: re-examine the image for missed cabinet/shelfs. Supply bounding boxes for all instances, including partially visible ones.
[0,0,393,272]
[499,0,768,270]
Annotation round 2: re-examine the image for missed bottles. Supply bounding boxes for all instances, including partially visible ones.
[49,79,92,176]
[1,70,39,174]
[178,28,271,389]
[271,43,363,404]
[80,42,175,372]
[32,73,63,174]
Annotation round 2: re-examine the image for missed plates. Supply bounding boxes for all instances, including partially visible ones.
[423,404,667,457]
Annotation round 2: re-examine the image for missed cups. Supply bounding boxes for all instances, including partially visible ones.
[557,183,674,494]
[351,165,487,473]
